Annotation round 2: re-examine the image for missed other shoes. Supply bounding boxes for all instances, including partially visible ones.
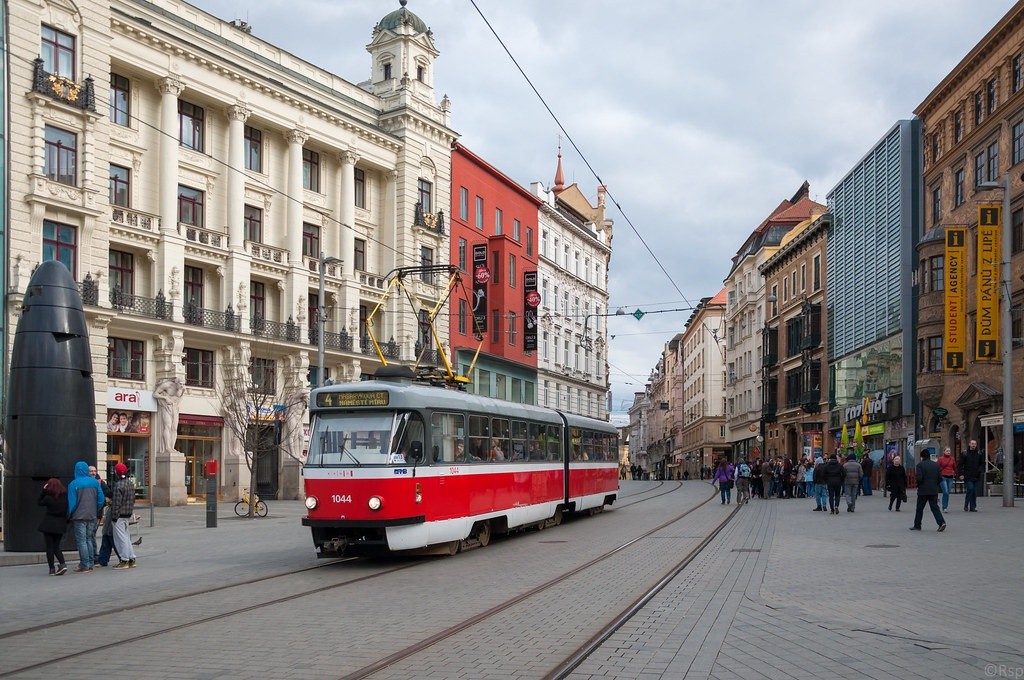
[943,508,948,512]
[964,506,968,511]
[823,506,827,511]
[96,563,106,568]
[830,512,834,514]
[847,503,852,512]
[888,507,891,511]
[896,509,900,511]
[835,508,839,514]
[910,527,920,530]
[113,559,136,570]
[970,509,977,512]
[738,494,815,504]
[813,507,822,511]
[73,566,93,573]
[937,522,946,531]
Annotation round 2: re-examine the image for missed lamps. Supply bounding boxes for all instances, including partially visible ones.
[768,294,777,302]
[617,307,624,315]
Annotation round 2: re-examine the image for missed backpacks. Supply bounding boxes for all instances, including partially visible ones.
[740,464,750,477]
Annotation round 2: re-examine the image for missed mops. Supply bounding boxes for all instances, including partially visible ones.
[985,459,1003,480]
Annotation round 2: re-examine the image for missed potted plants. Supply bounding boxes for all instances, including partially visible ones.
[989,471,1003,494]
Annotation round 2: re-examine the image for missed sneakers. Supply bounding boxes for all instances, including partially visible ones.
[49,569,55,575]
[56,564,67,575]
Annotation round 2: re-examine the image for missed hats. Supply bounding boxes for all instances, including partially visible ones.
[920,449,930,457]
[115,463,127,477]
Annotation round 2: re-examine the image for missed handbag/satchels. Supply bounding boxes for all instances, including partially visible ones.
[728,480,734,488]
[901,490,907,503]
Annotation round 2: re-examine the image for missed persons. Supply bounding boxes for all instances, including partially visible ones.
[630,464,643,480]
[712,453,873,514]
[909,448,946,531]
[937,447,957,513]
[621,464,626,480]
[38,462,136,576]
[383,433,409,462]
[700,465,711,480]
[958,439,984,512]
[684,470,689,479]
[677,470,680,479]
[886,456,907,511]
[107,412,137,433]
[454,434,553,460]
[573,441,608,460]
